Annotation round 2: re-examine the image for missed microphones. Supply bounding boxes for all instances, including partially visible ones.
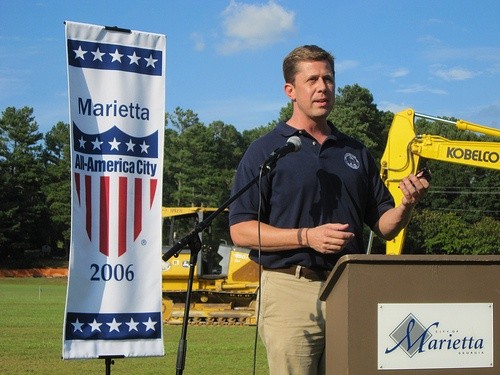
[263,136,302,163]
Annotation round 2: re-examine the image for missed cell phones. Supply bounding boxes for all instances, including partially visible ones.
[408,167,433,188]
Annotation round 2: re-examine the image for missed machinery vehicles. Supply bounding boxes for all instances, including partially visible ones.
[162,107,500,326]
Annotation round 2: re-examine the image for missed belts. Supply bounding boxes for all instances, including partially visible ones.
[263,262,331,281]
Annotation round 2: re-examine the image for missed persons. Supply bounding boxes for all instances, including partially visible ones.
[229,45,430,375]
